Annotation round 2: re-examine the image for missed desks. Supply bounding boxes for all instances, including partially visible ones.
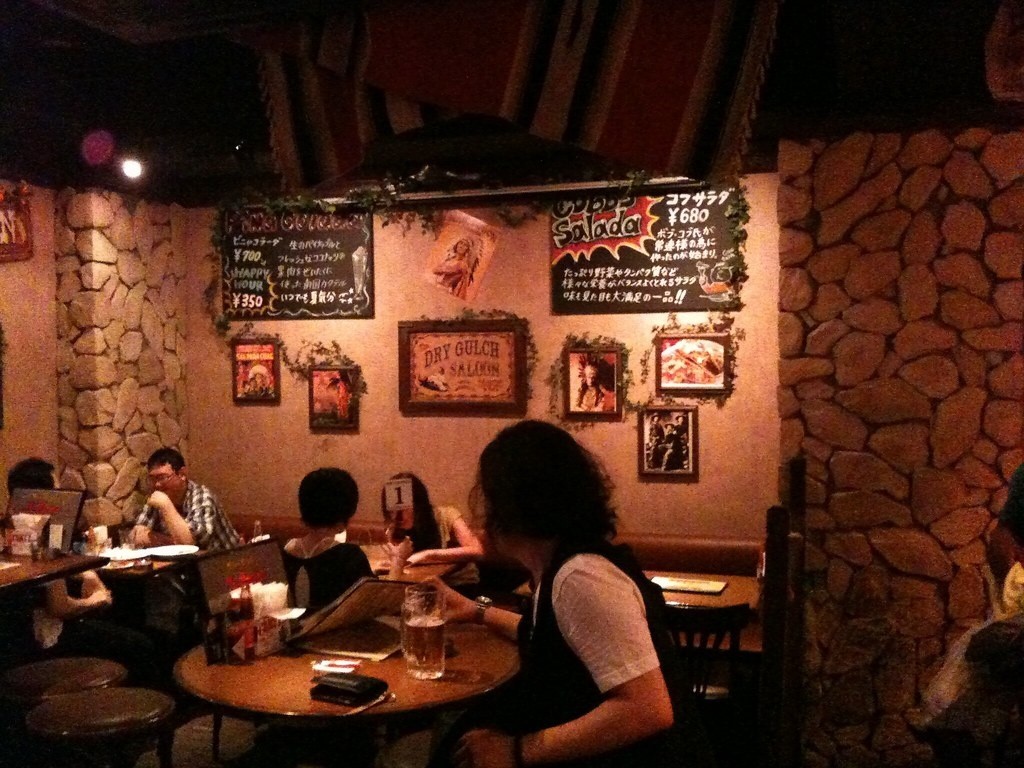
[174,614,520,768]
[514,570,759,623]
[96,551,209,624]
[366,555,458,582]
[0,552,110,593]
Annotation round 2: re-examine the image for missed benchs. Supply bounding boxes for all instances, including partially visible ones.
[229,514,764,655]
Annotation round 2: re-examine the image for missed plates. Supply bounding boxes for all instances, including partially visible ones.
[98,545,200,561]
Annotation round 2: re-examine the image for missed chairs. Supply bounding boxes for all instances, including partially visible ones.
[663,603,751,730]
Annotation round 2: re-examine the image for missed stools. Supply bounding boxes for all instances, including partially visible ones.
[0,657,128,722]
[26,687,176,768]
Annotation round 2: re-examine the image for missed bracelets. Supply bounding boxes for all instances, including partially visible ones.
[513,733,528,768]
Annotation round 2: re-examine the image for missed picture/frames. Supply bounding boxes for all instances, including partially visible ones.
[397,318,529,418]
[230,338,282,406]
[637,406,697,479]
[564,347,621,422]
[308,364,359,432]
[654,333,732,396]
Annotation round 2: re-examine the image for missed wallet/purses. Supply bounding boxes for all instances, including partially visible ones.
[310,672,387,706]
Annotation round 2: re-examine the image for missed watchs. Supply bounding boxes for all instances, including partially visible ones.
[473,595,493,625]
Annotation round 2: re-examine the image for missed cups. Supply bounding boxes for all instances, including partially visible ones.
[402,584,447,679]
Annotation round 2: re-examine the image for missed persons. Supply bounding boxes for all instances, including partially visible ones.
[646,411,689,471]
[7,457,168,691]
[432,234,479,301]
[236,358,275,399]
[111,445,239,653]
[280,468,414,621]
[381,471,486,602]
[426,419,718,768]
[986,461,1024,610]
[574,353,615,412]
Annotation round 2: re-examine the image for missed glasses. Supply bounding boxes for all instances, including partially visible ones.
[148,471,177,483]
